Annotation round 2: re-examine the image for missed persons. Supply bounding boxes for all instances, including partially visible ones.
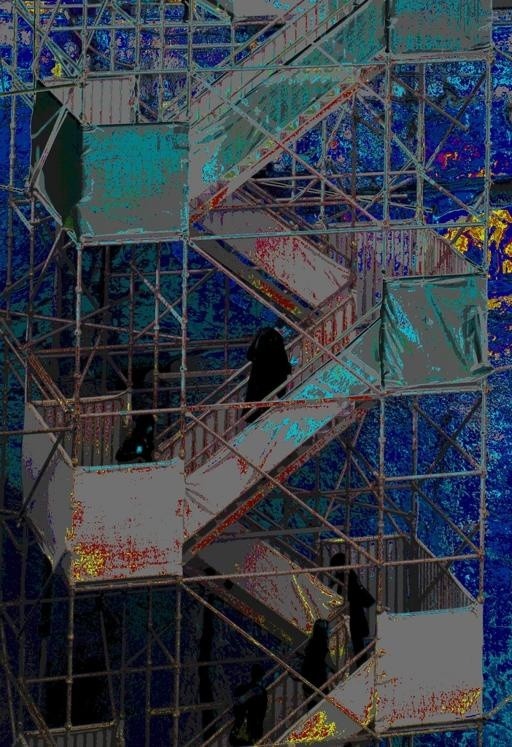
[231,657,267,747]
[302,619,328,710]
[115,414,147,460]
[330,553,376,670]
[244,325,293,415]
